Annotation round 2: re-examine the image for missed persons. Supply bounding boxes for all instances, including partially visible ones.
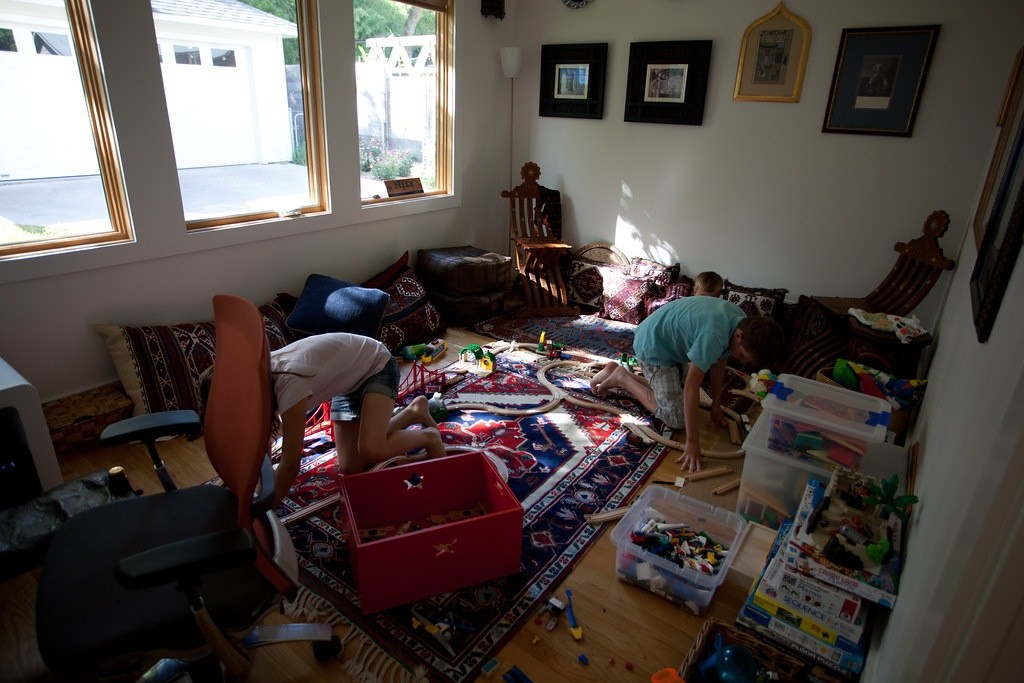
[590,296,784,473]
[197,332,447,504]
[693,272,724,297]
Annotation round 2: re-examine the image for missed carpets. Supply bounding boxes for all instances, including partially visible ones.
[474,311,641,365]
[208,340,674,683]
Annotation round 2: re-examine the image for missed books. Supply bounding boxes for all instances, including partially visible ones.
[734,468,911,680]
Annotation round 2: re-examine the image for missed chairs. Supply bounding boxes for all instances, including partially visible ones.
[504,163,571,317]
[786,210,961,378]
[34,293,342,683]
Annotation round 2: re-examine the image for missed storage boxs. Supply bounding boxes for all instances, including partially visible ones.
[337,453,527,619]
[610,373,908,680]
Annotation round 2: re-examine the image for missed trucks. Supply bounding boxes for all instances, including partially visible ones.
[421,337,448,366]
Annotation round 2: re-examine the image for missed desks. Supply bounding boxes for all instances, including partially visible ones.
[416,244,512,315]
[846,313,932,380]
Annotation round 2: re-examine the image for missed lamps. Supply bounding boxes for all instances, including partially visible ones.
[499,47,523,286]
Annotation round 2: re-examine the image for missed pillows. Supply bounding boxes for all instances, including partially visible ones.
[92,298,291,446]
[282,248,439,362]
[569,254,789,323]
[285,272,390,343]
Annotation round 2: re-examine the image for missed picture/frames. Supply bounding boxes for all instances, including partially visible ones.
[624,40,713,126]
[822,22,942,137]
[538,40,607,120]
[970,121,1024,343]
[732,0,812,103]
